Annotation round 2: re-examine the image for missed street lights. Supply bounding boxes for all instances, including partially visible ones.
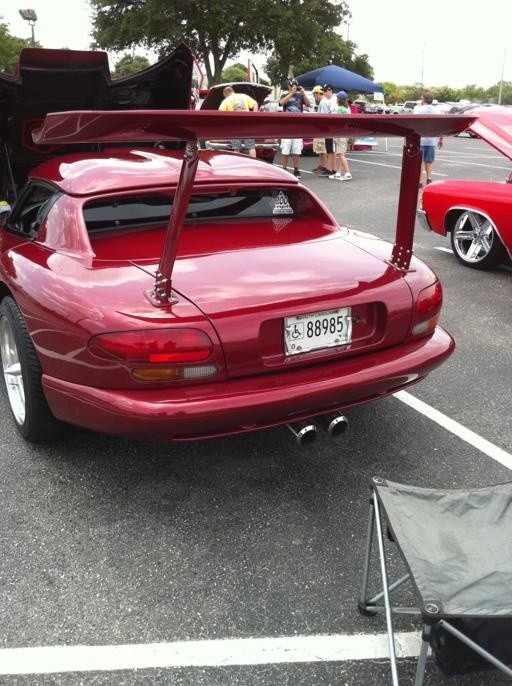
[19,8,38,41]
[344,20,350,40]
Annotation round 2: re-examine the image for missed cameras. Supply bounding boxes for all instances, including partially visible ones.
[296,86,301,91]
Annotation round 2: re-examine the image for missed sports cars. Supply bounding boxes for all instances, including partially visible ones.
[0,109,481,446]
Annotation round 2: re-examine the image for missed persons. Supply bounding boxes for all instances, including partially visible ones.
[219,87,258,159]
[279,82,310,179]
[414,92,443,188]
[312,85,359,181]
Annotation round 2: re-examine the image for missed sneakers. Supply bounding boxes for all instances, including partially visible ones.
[419,179,431,188]
[312,166,336,176]
[328,172,353,181]
[294,171,302,180]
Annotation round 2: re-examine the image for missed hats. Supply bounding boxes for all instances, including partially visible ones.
[287,78,298,86]
[320,84,333,91]
[336,91,348,100]
[312,86,324,94]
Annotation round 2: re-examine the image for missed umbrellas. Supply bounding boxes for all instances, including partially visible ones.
[279,65,384,94]
